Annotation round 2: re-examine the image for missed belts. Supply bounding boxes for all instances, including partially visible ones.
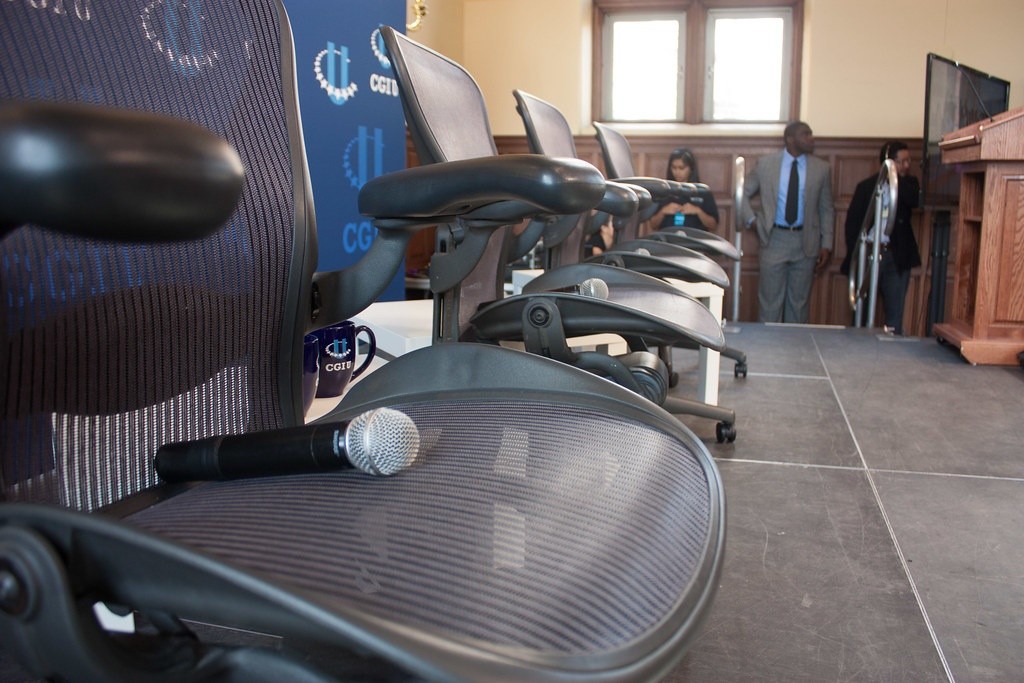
[773,223,803,231]
[867,242,892,249]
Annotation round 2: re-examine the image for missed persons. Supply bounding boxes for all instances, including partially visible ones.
[585,214,615,257]
[650,148,720,234]
[733,122,834,324]
[840,141,922,335]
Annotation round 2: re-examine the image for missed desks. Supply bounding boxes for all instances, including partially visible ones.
[405,277,431,300]
[511,268,725,409]
[303,299,630,426]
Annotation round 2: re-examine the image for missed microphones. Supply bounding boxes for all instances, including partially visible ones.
[154,407,421,483]
[477,278,608,312]
[634,248,651,256]
[674,231,686,237]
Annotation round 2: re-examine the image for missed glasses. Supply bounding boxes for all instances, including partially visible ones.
[894,157,911,164]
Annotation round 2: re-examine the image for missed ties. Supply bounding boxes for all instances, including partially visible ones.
[785,159,798,225]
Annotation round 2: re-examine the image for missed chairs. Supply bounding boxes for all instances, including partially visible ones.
[1,2,750,683]
